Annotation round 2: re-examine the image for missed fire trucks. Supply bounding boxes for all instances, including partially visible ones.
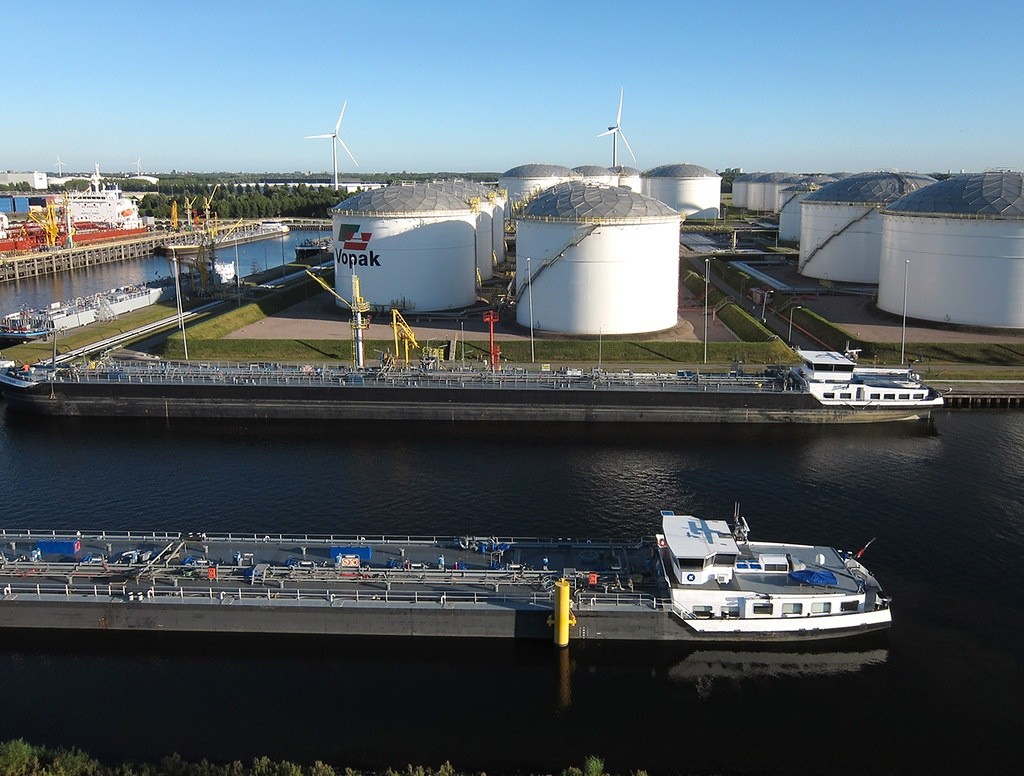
[747,287,773,306]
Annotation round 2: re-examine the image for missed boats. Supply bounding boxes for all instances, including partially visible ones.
[1,525,893,645]
[0,261,235,346]
[295,236,333,260]
[160,221,290,257]
[0,176,148,253]
[0,349,944,425]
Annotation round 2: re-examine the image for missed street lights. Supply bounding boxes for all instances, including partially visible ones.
[761,290,774,320]
[527,258,534,363]
[901,260,911,364]
[722,267,731,295]
[704,259,708,362]
[789,306,801,343]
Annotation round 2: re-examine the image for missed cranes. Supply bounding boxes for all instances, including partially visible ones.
[306,270,370,367]
[203,185,219,226]
[185,196,199,227]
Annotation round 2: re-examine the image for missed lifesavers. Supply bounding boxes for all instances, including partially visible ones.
[659,539,666,546]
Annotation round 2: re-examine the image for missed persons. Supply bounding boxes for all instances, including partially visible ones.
[542,556,549,570]
[438,553,459,570]
[7,362,31,374]
[34,549,41,561]
[101,558,110,574]
